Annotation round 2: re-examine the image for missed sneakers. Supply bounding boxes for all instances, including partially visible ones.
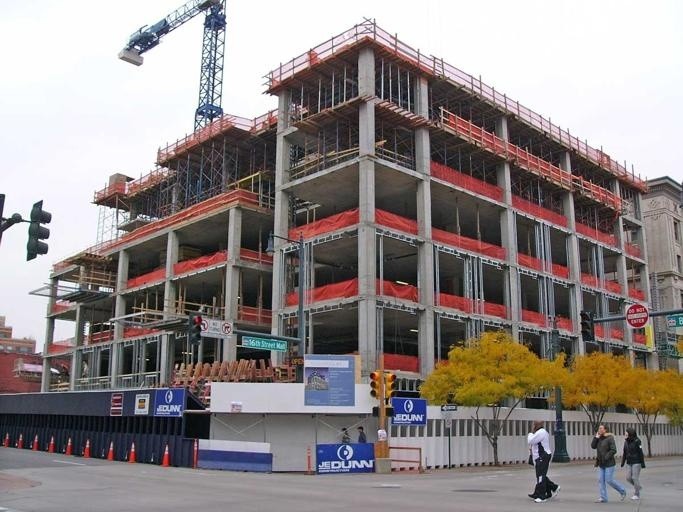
[621,490,626,501]
[631,496,639,500]
[529,486,561,503]
[597,499,606,502]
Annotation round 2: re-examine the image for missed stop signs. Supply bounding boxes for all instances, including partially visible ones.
[626,303,648,329]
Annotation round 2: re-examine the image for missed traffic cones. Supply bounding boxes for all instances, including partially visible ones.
[126,442,136,463]
[82,439,93,458]
[160,445,171,468]
[105,441,116,462]
[2,432,73,456]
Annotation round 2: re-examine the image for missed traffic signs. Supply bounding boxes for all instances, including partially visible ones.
[440,404,457,412]
[242,335,289,353]
[666,314,683,328]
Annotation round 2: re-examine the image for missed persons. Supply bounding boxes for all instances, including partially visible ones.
[621,427,646,500]
[591,423,627,503]
[341,428,350,444]
[527,421,552,501]
[526,418,561,503]
[356,426,366,443]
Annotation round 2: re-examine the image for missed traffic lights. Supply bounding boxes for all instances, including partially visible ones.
[371,406,379,417]
[369,370,381,397]
[580,309,595,341]
[188,314,203,347]
[385,373,396,398]
[26,201,53,260]
[384,406,396,417]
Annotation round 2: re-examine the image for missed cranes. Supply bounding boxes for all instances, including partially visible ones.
[115,0,225,142]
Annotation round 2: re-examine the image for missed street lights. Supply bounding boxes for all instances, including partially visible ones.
[266,230,307,385]
[545,314,571,463]
